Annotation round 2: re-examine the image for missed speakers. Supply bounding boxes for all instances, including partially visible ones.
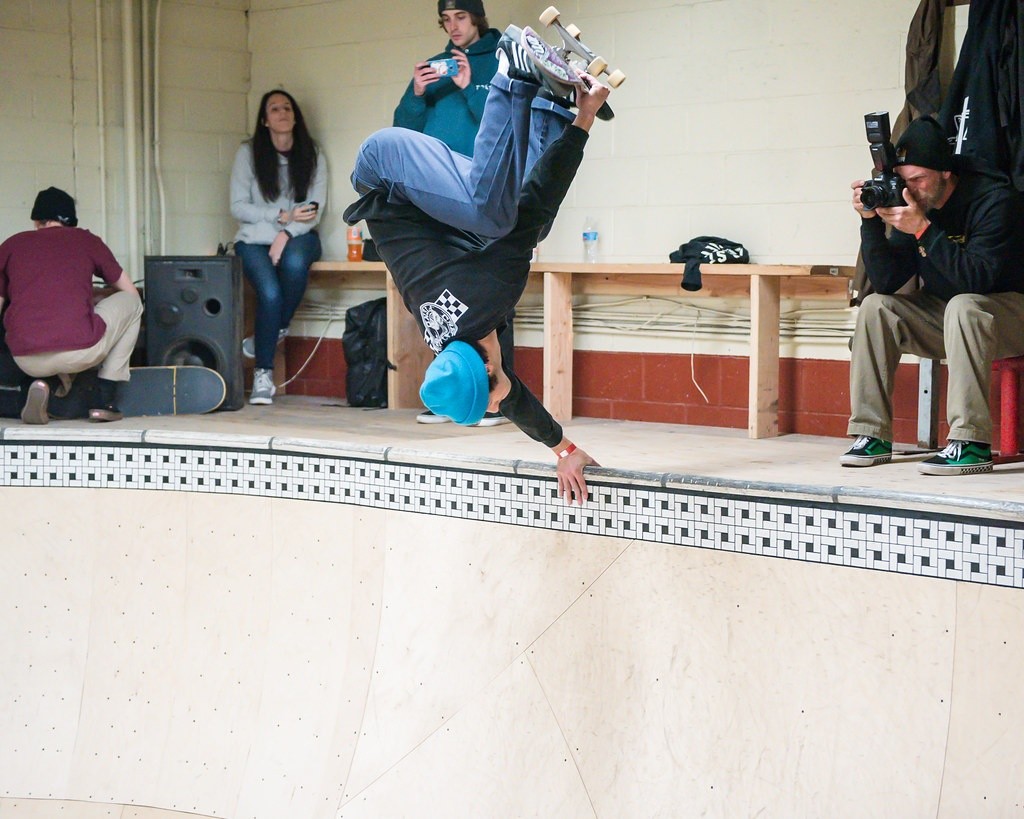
[143,254,245,410]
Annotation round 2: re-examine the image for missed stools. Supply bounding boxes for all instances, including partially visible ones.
[938,354,1024,464]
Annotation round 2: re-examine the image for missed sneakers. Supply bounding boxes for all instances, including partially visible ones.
[917,440,993,476]
[243,328,286,358]
[249,368,275,404]
[839,434,893,467]
[495,24,545,85]
[542,74,573,97]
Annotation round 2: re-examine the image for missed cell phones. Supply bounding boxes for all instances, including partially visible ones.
[426,58,458,77]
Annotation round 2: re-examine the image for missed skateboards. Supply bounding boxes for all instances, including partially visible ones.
[520,5,627,122]
[19,364,228,421]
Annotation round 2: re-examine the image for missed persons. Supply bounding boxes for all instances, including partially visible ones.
[229,91,327,403]
[342,24,610,505]
[392,0,514,427]
[0,187,143,424]
[839,116,1024,475]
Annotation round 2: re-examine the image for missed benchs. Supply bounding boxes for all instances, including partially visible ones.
[238,256,860,437]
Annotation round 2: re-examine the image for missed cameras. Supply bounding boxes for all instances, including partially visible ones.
[859,111,908,209]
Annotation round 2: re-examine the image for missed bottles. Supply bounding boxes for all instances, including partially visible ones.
[529,243,537,262]
[582,215,599,263]
[346,225,363,262]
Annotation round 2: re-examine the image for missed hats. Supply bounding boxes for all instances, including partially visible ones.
[893,115,955,172]
[418,341,489,425]
[30,186,79,227]
[438,0,486,17]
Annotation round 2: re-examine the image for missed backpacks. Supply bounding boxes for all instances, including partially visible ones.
[343,298,398,407]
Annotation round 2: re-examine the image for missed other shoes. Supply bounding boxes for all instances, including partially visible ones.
[89,396,123,421]
[478,412,512,426]
[416,411,451,423]
[21,379,49,424]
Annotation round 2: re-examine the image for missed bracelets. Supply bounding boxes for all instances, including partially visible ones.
[278,209,282,224]
[915,224,929,240]
[284,229,292,238]
[557,443,576,458]
